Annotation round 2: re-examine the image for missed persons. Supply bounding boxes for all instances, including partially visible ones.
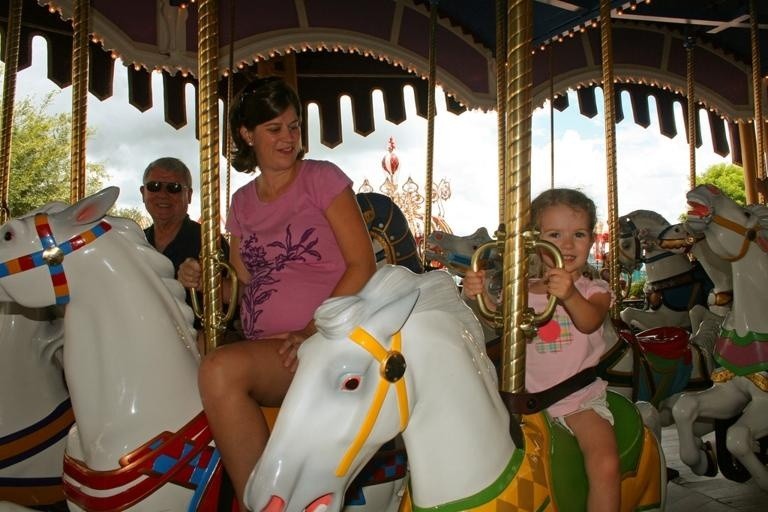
[196,76,378,512]
[138,157,229,332]
[460,186,622,511]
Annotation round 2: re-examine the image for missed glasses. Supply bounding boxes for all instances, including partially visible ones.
[145,181,189,195]
